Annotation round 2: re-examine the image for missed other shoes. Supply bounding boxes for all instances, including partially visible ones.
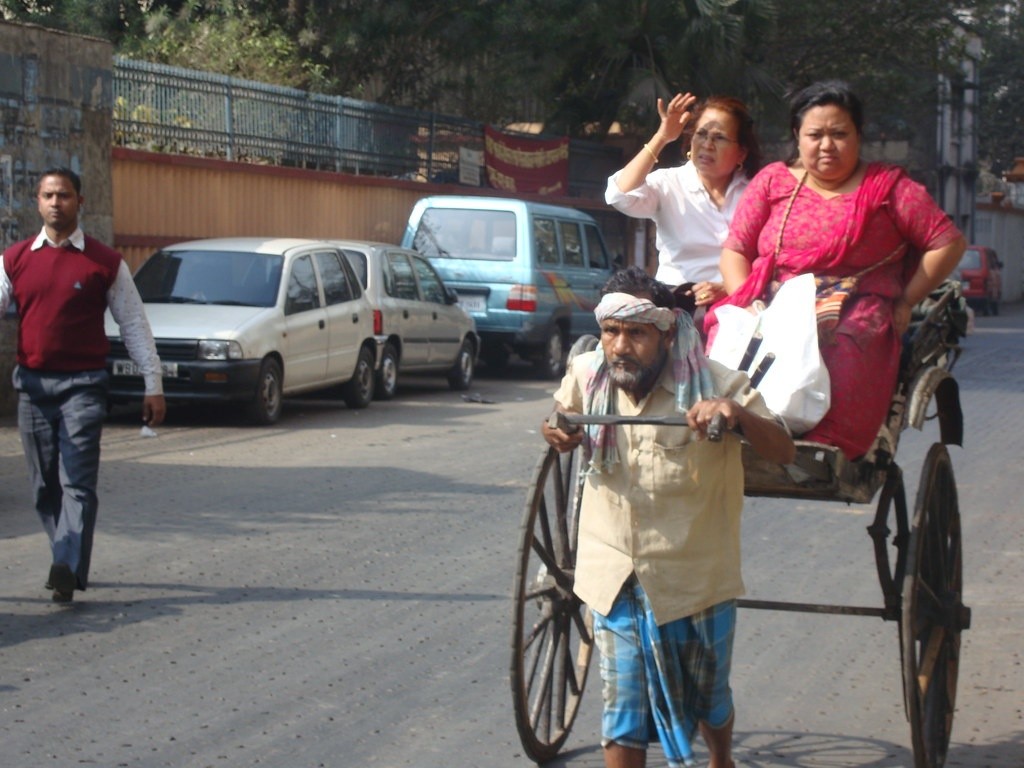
[45,560,75,603]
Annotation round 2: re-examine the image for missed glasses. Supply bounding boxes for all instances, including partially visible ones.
[684,128,741,144]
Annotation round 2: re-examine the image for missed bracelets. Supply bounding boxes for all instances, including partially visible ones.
[645,144,659,163]
[902,296,914,307]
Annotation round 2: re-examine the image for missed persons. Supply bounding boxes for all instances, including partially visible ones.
[604,92,760,342]
[705,79,965,461]
[540,267,795,768]
[0,166,166,600]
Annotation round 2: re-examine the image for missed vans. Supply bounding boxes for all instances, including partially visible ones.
[399,196,626,380]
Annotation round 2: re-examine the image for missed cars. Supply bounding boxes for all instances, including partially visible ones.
[330,239,481,400]
[101,237,378,427]
[945,245,1006,316]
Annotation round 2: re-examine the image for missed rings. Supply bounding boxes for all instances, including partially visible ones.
[701,294,707,299]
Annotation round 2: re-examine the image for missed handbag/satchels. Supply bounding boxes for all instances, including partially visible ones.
[709,271,831,436]
[765,274,864,340]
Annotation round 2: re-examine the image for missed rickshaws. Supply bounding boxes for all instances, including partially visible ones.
[506,272,973,766]
